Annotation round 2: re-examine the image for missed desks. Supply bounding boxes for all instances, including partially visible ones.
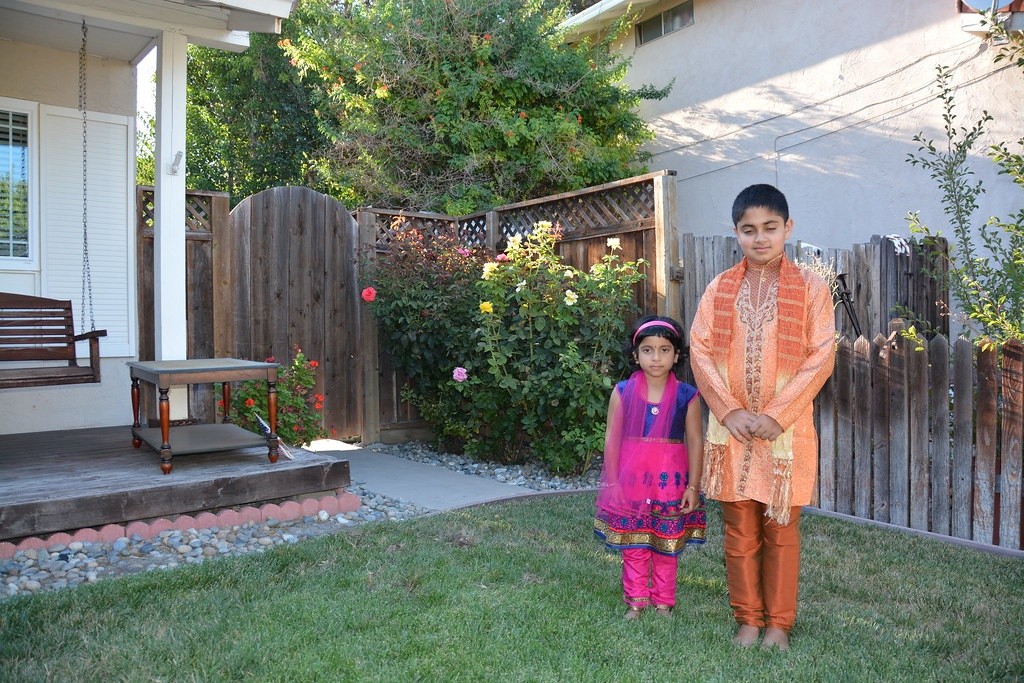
[126,358,282,475]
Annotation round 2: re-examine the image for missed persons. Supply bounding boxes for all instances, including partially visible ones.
[690,184,836,650]
[592,317,705,618]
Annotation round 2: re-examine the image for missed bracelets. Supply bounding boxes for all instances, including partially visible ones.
[686,485,700,491]
[597,481,616,487]
